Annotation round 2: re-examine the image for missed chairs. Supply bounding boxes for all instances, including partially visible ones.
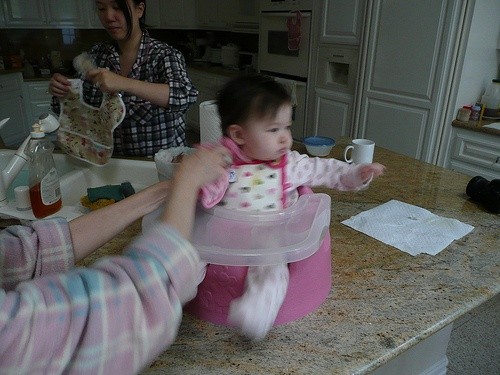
[142,175,331,333]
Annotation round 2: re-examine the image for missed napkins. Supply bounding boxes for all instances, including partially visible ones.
[340,199,472,257]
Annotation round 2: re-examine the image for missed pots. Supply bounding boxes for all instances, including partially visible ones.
[222,42,240,67]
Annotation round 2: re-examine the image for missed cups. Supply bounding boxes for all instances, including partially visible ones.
[344,139,375,165]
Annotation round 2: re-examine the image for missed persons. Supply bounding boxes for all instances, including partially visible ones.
[49,0,199,159]
[197,73,391,341]
[0,148,225,375]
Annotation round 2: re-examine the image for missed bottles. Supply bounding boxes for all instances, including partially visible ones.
[457,106,472,122]
[24,123,62,219]
[472,100,480,120]
[482,79,500,117]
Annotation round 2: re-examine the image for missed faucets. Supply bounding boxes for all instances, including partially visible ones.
[1,113,60,195]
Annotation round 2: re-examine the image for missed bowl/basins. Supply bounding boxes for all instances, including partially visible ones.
[303,136,334,157]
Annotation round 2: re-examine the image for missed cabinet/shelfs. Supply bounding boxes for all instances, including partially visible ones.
[186,70,224,127]
[258,0,500,182]
[24,82,53,123]
[0,72,30,147]
[0,0,259,34]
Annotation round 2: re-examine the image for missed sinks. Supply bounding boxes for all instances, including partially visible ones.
[0,144,70,200]
[56,158,160,219]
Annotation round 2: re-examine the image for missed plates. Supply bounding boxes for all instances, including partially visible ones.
[208,48,222,63]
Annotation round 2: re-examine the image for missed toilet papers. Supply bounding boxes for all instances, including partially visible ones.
[198,100,223,143]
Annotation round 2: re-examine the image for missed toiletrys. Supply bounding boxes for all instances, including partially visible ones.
[28,119,62,220]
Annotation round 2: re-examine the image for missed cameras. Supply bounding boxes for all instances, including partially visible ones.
[466,176,500,215]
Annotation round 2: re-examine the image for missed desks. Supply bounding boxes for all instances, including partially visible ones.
[0,142,500,375]
